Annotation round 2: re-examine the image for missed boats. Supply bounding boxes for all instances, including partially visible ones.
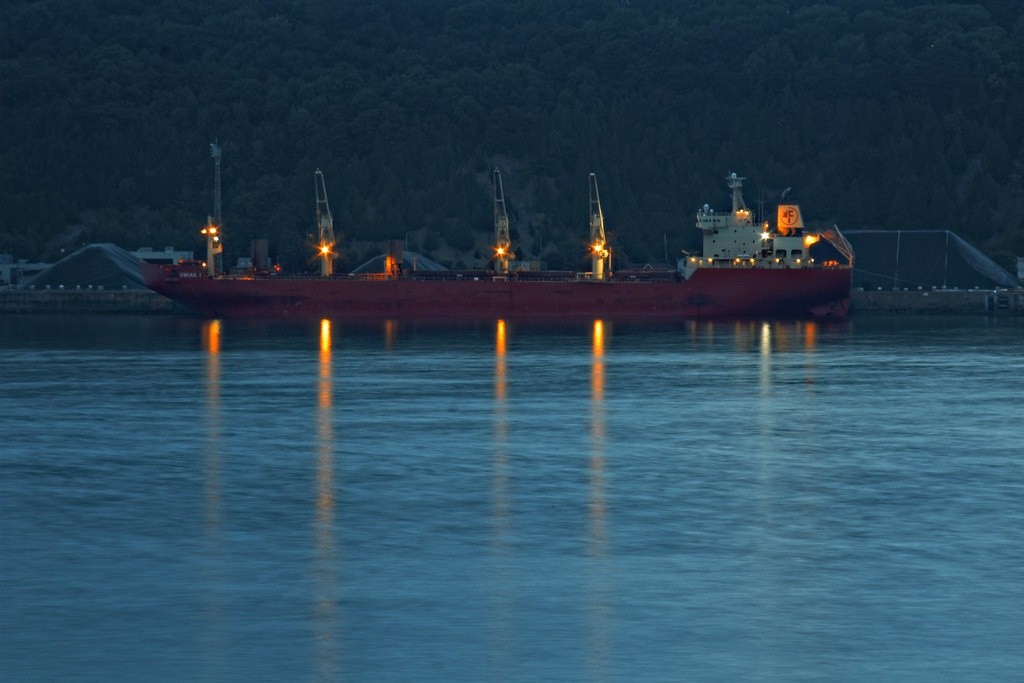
[139,169,855,321]
